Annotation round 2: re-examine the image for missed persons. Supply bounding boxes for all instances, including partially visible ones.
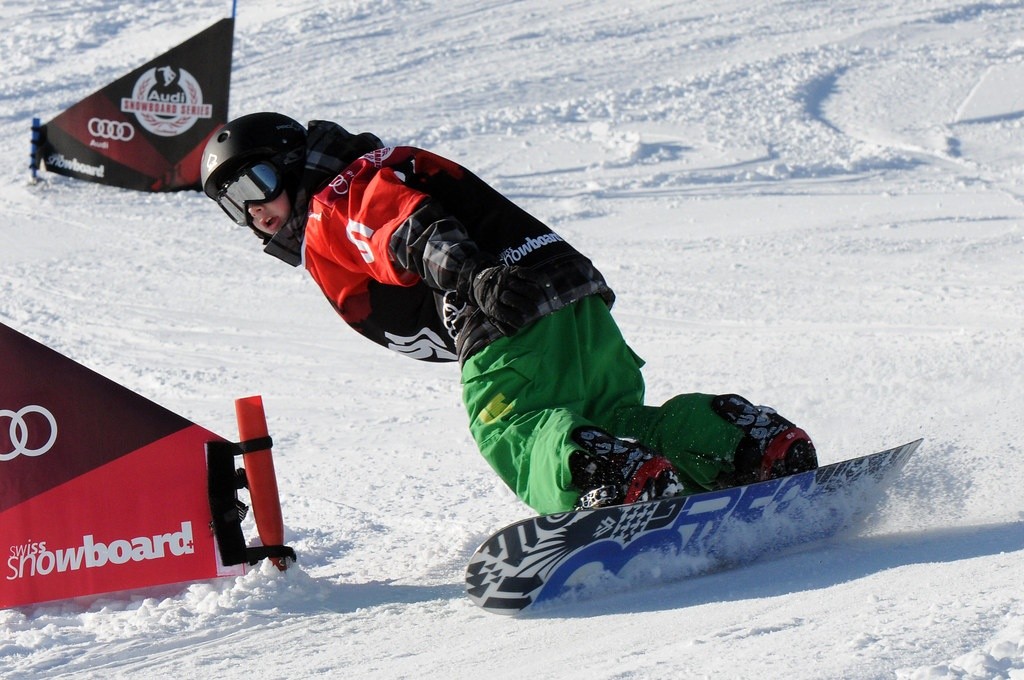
[199,112,822,513]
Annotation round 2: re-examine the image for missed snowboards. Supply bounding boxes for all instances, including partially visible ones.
[463,437,923,619]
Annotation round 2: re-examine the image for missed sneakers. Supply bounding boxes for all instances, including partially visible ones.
[710,393,818,485]
[569,423,685,512]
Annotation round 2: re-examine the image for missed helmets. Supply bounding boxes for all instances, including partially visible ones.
[200,112,308,200]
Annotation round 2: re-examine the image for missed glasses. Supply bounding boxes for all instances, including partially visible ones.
[213,160,285,227]
